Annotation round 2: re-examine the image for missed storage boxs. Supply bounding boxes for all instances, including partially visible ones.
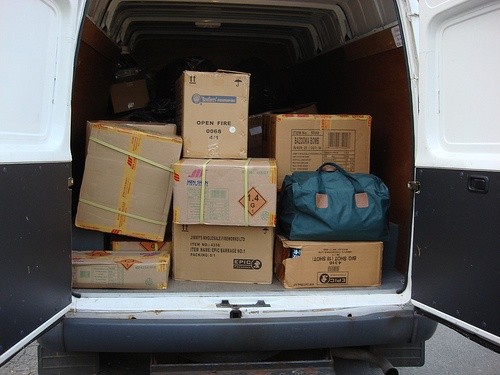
[75,122,183,243]
[273,103,318,114]
[172,224,275,285]
[110,80,150,113]
[268,113,373,192]
[85,119,177,162]
[175,68,251,160]
[249,112,273,149]
[73,250,170,289]
[170,158,278,229]
[275,234,384,289]
[111,240,172,253]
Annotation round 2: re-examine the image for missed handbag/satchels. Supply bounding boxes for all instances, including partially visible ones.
[277,163,390,240]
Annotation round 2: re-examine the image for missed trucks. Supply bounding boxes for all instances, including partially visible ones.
[0,0,500,375]
